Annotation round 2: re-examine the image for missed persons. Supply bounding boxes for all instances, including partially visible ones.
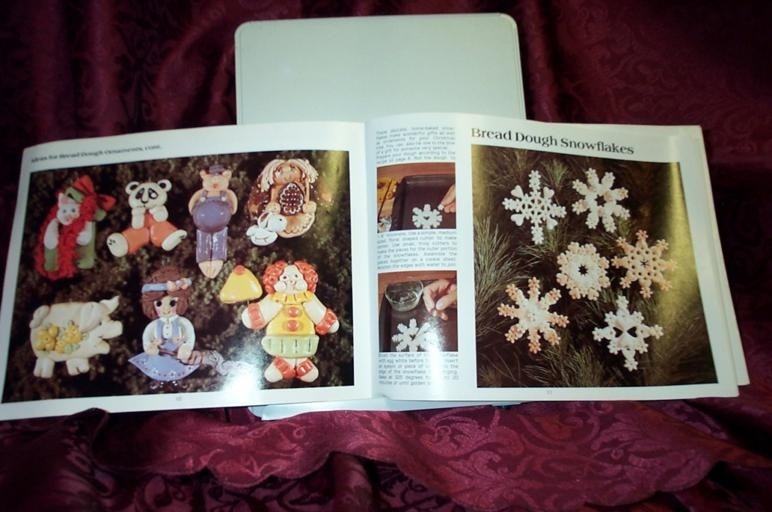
[421,279,457,319]
[438,186,455,212]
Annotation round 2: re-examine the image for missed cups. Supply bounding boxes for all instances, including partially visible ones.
[378,214,392,231]
[384,276,424,312]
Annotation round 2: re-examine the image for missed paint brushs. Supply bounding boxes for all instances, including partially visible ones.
[412,275,455,341]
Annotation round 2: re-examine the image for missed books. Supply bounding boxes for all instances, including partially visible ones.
[1,113,749,422]
